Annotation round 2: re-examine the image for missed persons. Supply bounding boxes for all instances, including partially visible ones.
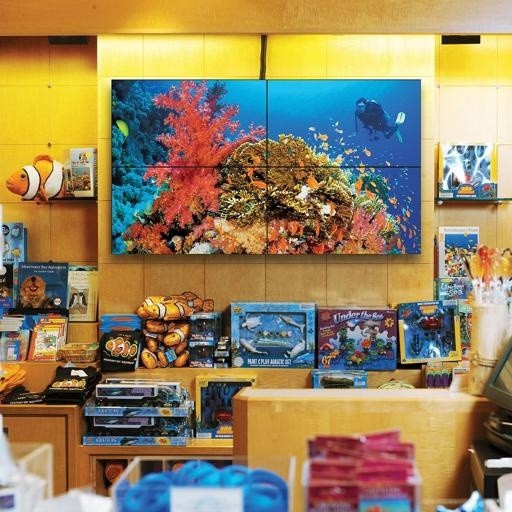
[356,97,406,146]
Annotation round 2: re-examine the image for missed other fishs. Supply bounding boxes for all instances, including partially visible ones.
[252,121,421,255]
[116,119,130,139]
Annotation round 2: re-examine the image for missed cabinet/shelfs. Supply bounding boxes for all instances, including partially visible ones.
[0,361,93,498]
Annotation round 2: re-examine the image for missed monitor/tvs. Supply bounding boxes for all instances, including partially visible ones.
[483,334,512,434]
